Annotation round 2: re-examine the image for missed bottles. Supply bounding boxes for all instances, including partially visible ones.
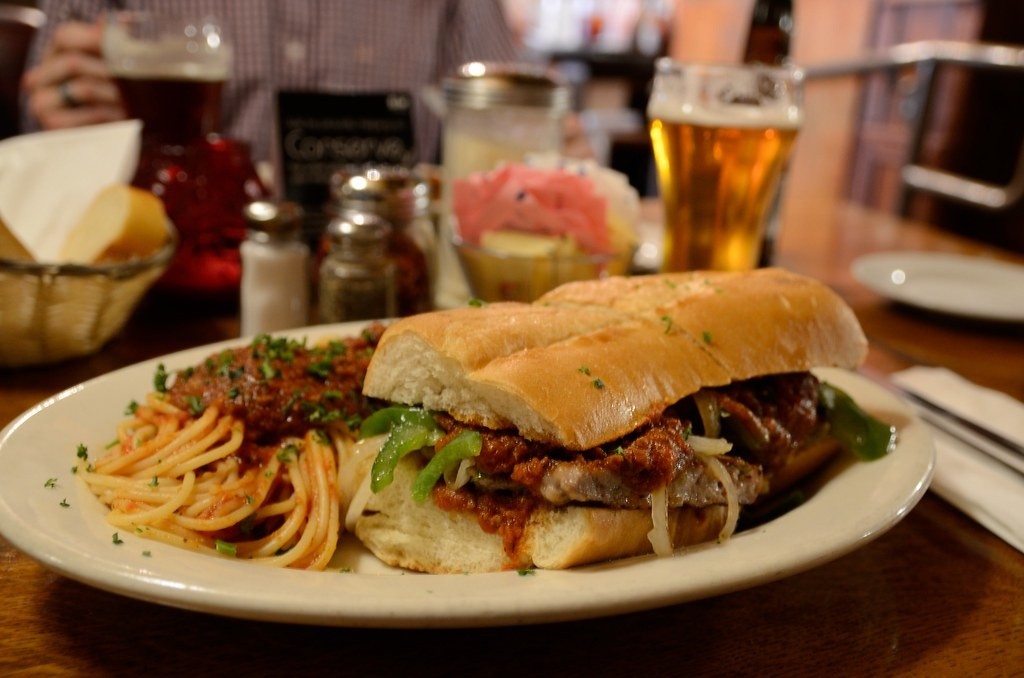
[241,176,432,337]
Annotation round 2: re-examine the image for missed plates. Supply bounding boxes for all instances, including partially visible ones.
[0,319,922,629]
[849,252,1024,325]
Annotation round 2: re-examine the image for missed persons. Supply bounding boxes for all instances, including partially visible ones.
[19,1,594,167]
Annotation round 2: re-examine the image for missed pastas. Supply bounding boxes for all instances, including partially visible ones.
[82,391,341,572]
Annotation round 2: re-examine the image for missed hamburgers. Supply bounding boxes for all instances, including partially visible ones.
[642,392,646,396]
[351,269,871,574]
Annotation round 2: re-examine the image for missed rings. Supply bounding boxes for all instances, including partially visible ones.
[59,77,82,109]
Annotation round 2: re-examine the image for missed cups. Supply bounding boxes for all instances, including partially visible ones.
[645,57,805,273]
[102,7,232,143]
[434,62,564,310]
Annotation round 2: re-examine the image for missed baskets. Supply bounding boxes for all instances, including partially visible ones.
[1,216,180,368]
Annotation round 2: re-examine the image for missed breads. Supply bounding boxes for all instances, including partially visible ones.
[0,184,172,263]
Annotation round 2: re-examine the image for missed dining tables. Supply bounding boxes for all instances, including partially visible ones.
[0,193,1024,678]
